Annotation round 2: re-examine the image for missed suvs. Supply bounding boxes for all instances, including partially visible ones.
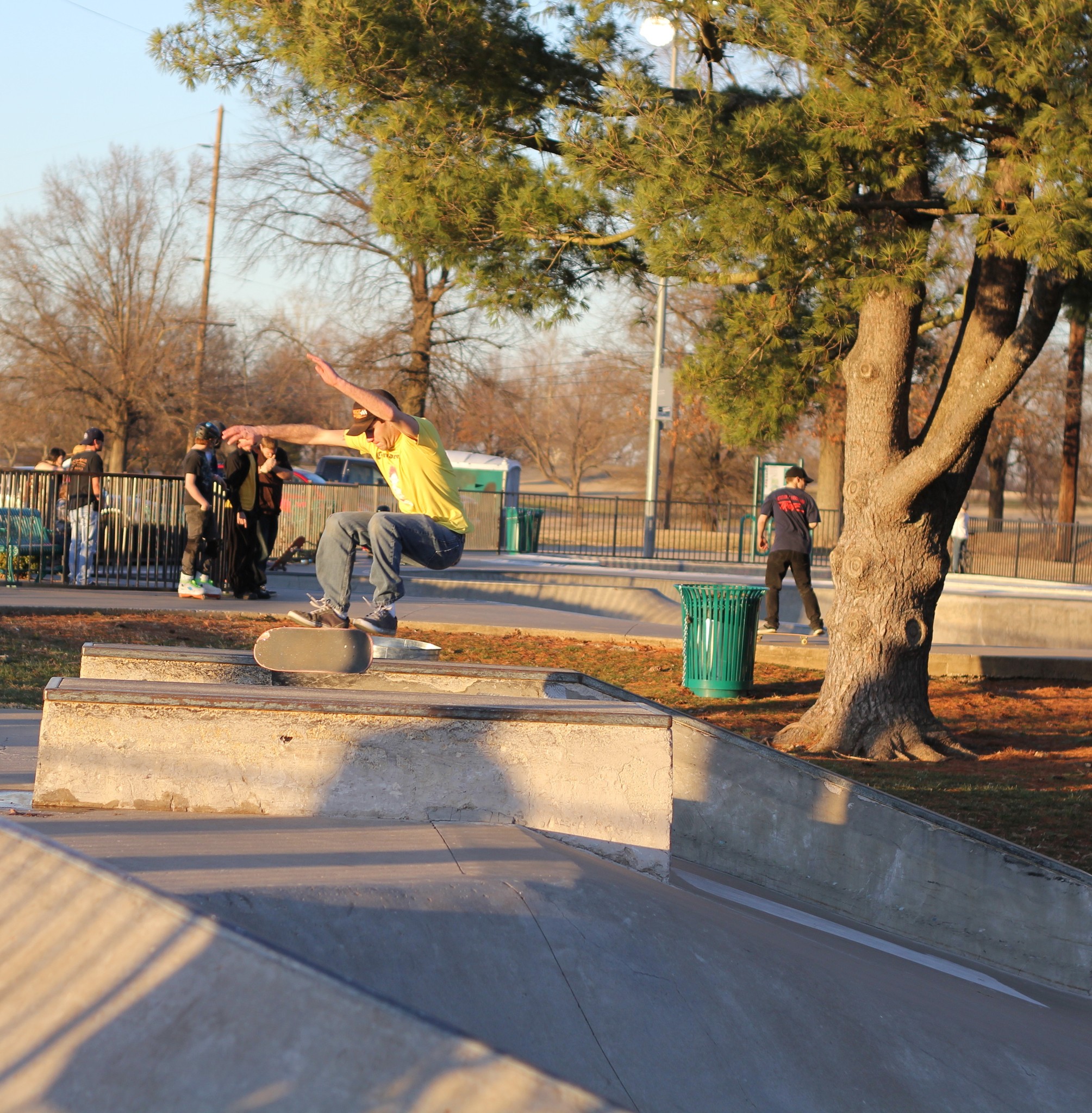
[315,454,388,486]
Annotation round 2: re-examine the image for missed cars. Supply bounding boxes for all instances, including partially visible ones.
[215,464,332,514]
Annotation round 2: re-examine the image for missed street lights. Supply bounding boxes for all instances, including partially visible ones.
[640,13,678,558]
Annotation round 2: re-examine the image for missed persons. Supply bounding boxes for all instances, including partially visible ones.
[950,500,969,573]
[29,427,104,585]
[178,421,292,600]
[754,466,826,635]
[218,354,470,638]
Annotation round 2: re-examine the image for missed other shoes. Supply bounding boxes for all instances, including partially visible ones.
[813,628,826,634]
[757,625,777,633]
[233,590,258,600]
[256,585,277,597]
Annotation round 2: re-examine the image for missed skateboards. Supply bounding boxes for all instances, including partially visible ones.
[268,537,306,572]
[755,631,823,644]
[254,627,374,676]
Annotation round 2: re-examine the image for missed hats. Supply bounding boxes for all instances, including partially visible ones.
[66,444,86,456]
[785,466,815,484]
[79,426,104,445]
[346,389,398,436]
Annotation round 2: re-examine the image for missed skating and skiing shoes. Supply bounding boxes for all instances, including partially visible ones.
[194,571,221,599]
[178,571,206,600]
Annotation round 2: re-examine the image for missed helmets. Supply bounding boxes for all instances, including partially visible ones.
[195,422,221,441]
[211,420,226,438]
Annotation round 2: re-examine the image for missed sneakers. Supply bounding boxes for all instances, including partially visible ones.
[287,593,350,629]
[353,596,397,636]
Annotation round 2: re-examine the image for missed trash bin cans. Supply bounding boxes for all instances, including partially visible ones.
[674,582,770,700]
[500,507,546,556]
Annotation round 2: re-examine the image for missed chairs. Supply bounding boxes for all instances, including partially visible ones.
[0,507,71,588]
